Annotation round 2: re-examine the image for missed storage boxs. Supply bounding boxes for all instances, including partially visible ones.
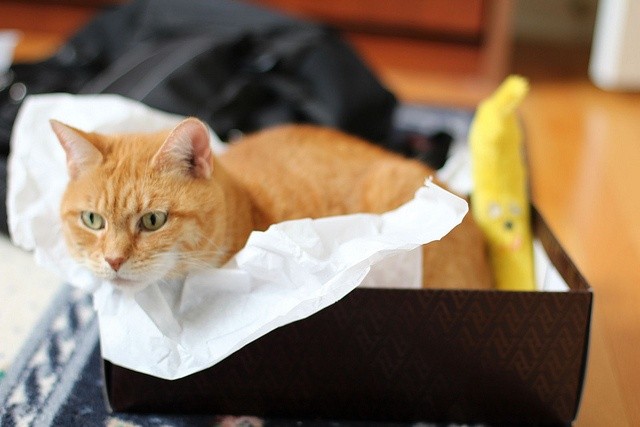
[100,187,592,426]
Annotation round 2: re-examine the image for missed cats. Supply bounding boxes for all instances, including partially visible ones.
[47,115,497,293]
